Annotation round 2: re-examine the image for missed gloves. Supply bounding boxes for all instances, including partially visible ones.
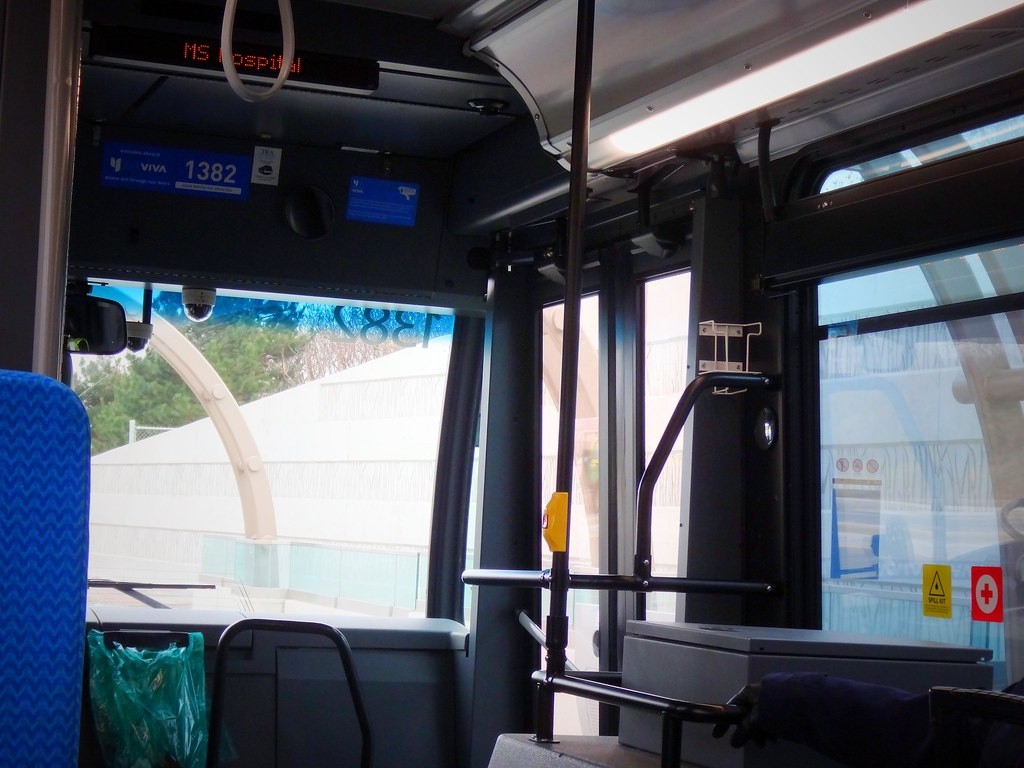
[711,684,778,749]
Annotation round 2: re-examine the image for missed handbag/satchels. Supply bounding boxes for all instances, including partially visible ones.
[86,628,208,768]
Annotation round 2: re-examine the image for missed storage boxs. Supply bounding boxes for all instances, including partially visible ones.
[617,618,996,768]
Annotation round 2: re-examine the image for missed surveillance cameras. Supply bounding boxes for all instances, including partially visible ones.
[182,287,216,323]
[126,321,153,352]
